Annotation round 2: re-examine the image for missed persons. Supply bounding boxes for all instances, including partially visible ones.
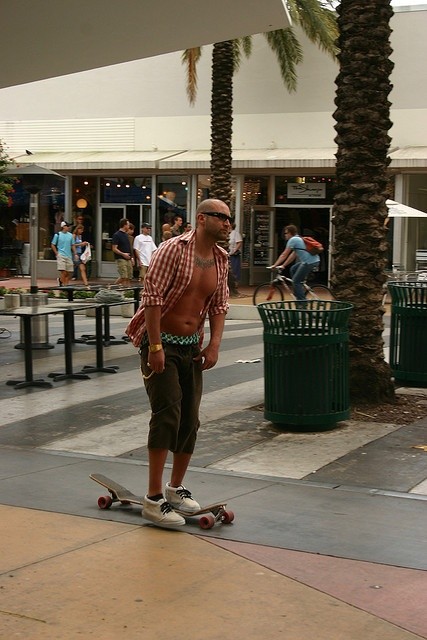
[162,232,172,240]
[133,223,158,278]
[228,224,243,289]
[112,218,132,278]
[126,223,139,278]
[72,215,84,229]
[171,217,183,236]
[124,199,234,529]
[269,225,321,311]
[161,223,170,231]
[51,219,75,285]
[73,226,94,286]
[183,222,191,233]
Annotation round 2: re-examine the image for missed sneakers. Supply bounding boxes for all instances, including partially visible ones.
[57,278,62,287]
[142,493,186,527]
[297,304,308,309]
[165,481,201,513]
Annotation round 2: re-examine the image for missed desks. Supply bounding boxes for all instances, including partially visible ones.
[36,281,144,345]
[44,301,96,382]
[0,299,69,388]
[79,293,138,374]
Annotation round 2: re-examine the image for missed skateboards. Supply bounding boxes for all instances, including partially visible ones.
[89,472,234,529]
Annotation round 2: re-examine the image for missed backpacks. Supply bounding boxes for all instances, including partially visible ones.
[294,236,324,255]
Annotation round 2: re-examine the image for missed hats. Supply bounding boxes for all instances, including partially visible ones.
[60,221,68,227]
[142,223,152,227]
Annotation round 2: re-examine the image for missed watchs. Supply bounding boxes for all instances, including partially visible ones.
[147,344,163,354]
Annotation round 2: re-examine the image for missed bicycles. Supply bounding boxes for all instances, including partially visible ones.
[252,265,335,319]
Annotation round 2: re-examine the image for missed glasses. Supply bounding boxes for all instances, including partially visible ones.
[284,233,289,234]
[146,227,151,229]
[79,218,83,220]
[201,212,233,224]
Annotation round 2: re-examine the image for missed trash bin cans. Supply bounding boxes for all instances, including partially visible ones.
[387,280,427,387]
[256,300,355,432]
